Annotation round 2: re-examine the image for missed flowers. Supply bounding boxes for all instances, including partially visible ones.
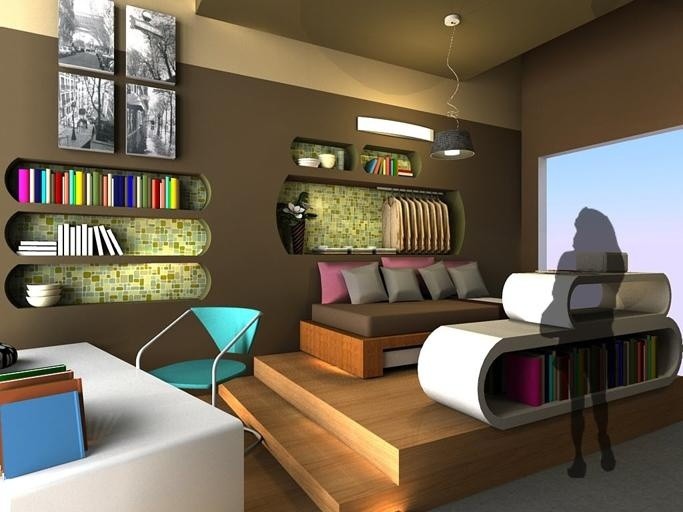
[277,191,317,226]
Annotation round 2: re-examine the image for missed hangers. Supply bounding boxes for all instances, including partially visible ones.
[389,188,440,198]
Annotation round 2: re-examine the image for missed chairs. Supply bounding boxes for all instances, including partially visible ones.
[136,307,262,453]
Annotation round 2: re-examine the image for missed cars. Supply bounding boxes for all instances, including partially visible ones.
[93,49,114,71]
[57,47,94,57]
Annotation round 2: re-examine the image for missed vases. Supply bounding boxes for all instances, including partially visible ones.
[291,221,305,255]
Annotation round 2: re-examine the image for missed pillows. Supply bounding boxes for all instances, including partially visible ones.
[317,262,374,305]
[380,266,424,304]
[444,262,464,267]
[418,260,456,301]
[381,256,435,275]
[447,261,489,299]
[342,263,388,305]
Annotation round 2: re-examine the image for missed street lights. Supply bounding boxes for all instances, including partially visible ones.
[70,101,77,140]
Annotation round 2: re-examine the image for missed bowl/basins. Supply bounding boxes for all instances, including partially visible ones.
[318,153,335,168]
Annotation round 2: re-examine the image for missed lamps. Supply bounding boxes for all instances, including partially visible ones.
[430,14,474,160]
[357,115,434,142]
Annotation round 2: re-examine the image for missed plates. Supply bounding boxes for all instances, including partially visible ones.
[297,157,320,168]
[24,282,64,309]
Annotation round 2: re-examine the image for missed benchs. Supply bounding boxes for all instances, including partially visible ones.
[300,300,503,378]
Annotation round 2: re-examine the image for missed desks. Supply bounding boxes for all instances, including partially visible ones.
[0,342,244,512]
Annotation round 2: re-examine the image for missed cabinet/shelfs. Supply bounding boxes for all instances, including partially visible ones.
[276,136,466,256]
[417,273,682,429]
[4,157,212,310]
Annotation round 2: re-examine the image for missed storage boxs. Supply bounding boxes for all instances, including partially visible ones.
[576,253,628,271]
